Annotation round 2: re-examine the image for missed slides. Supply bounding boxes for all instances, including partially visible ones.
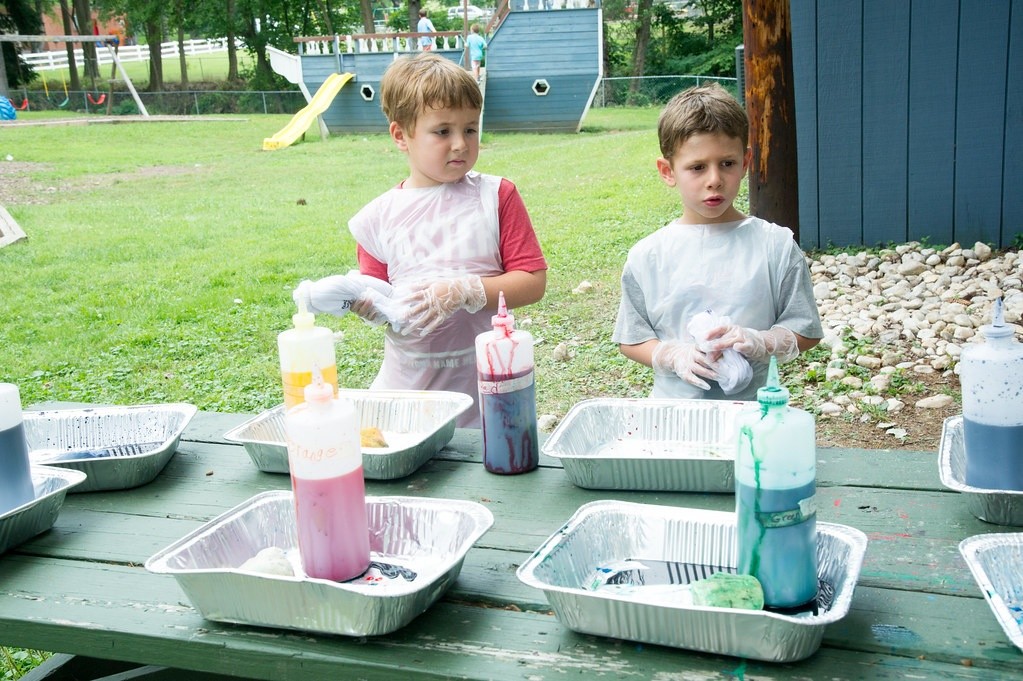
[261,71,357,152]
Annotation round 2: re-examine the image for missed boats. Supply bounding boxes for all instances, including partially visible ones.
[264,0,605,132]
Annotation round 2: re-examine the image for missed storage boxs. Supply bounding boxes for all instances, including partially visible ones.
[25,402,197,492]
[939,415,1023,526]
[0,464,88,554]
[959,532,1023,652]
[547,396,762,493]
[225,388,475,481]
[146,489,496,635]
[515,500,869,660]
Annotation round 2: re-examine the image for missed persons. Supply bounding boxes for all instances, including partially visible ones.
[464,24,487,85]
[415,9,437,51]
[609,83,825,403]
[344,54,547,429]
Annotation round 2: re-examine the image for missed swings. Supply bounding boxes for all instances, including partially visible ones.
[32,43,70,109]
[78,42,109,106]
[1,42,28,111]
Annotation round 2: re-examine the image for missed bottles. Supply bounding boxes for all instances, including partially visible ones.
[278,297,340,406]
[958,295,1022,495]
[732,356,820,611]
[285,364,372,583]
[474,291,541,476]
[0,381,37,514]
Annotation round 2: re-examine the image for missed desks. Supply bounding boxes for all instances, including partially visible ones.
[0,410,1023,681]
